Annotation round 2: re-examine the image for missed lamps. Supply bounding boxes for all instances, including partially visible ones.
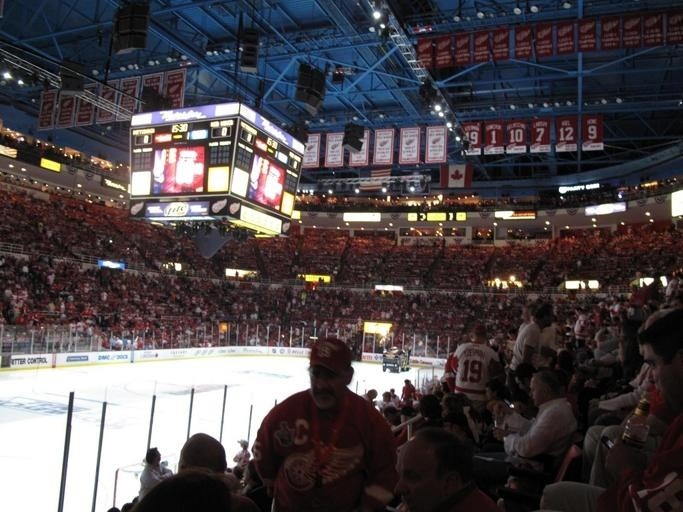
[368,0,389,37]
[446,119,458,132]
[455,128,463,142]
[429,96,445,118]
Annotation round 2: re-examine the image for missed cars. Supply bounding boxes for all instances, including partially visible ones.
[495,415,505,432]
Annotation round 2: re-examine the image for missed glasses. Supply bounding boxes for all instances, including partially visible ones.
[429,96,445,118]
[455,128,463,142]
[368,0,389,37]
[446,119,458,132]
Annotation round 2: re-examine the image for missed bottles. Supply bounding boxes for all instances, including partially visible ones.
[310,337,351,374]
[420,395,442,416]
[238,440,249,447]
[146,447,159,463]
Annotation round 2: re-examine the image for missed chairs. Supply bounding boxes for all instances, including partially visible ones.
[1,196,683,512]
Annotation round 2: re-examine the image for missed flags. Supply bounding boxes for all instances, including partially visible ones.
[439,165,472,188]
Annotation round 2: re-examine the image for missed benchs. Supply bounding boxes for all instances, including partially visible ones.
[439,165,472,188]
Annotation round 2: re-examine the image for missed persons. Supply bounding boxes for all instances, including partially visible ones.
[0,223,683,365]
[1,191,151,229]
[296,184,683,212]
[107,337,682,512]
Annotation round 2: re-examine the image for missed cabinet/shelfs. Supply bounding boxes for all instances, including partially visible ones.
[620,397,652,446]
